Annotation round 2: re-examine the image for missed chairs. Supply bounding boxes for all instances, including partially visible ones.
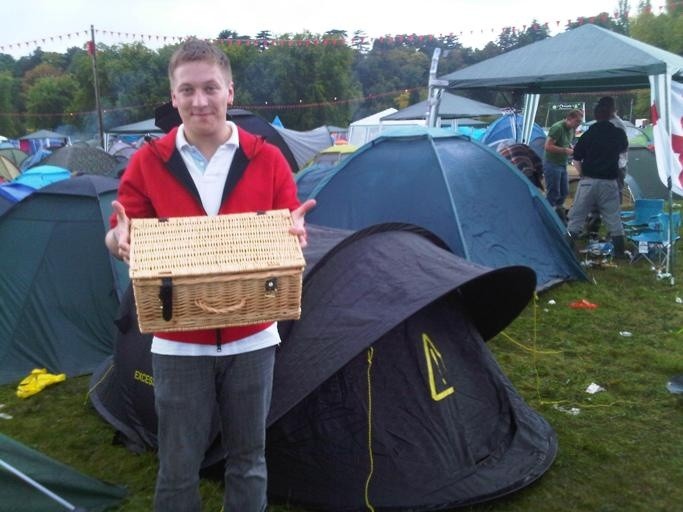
[615,199,681,280]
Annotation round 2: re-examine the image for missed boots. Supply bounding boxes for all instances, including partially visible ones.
[609,235,628,260]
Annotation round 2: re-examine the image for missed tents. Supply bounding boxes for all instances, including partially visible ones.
[624,121,682,202]
[88,221,556,512]
[301,128,596,292]
[0,96,508,511]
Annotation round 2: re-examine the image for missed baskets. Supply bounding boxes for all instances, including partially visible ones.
[128,206,308,335]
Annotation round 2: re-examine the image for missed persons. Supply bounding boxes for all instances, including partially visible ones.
[103,35,316,509]
[567,97,631,259]
[543,108,583,210]
